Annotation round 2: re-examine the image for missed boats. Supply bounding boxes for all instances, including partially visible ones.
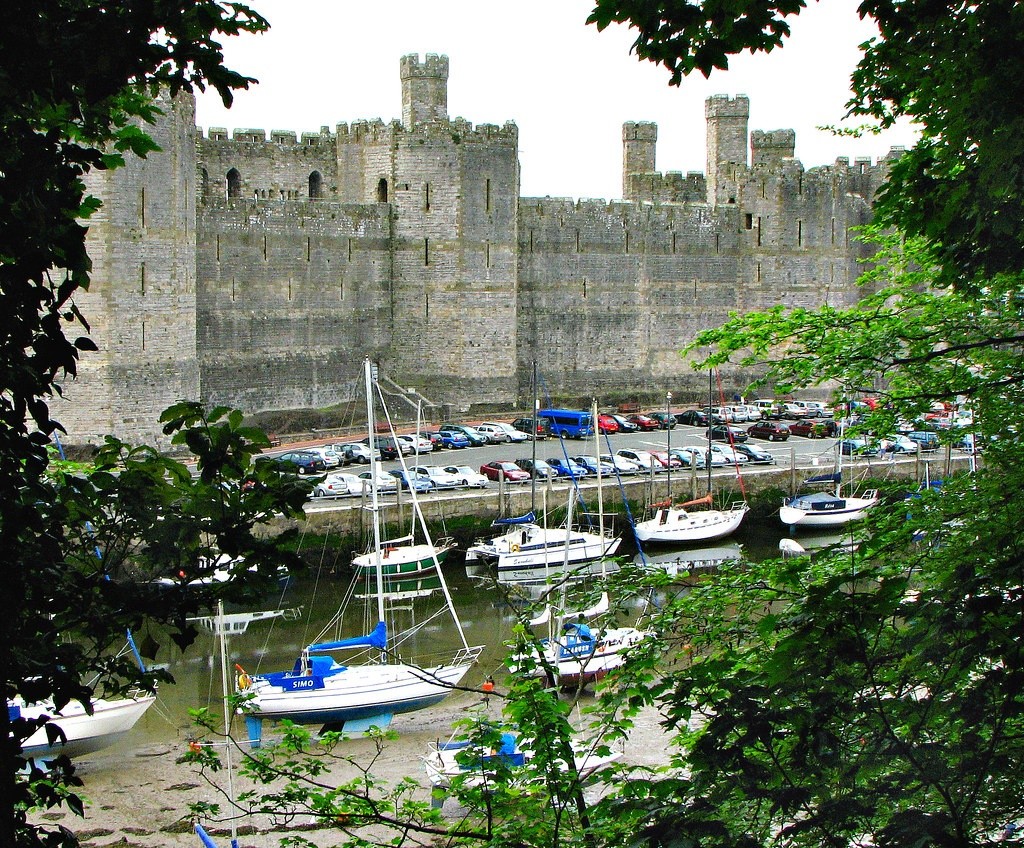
[5,678,158,765]
[421,729,625,790]
[121,548,298,601]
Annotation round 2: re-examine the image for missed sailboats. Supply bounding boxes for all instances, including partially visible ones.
[509,480,657,687]
[465,358,623,570]
[630,341,752,545]
[350,474,458,578]
[227,355,485,726]
[777,365,882,529]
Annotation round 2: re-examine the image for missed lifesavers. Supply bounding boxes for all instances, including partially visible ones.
[511,545,519,552]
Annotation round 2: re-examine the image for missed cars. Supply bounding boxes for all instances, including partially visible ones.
[255,391,990,502]
